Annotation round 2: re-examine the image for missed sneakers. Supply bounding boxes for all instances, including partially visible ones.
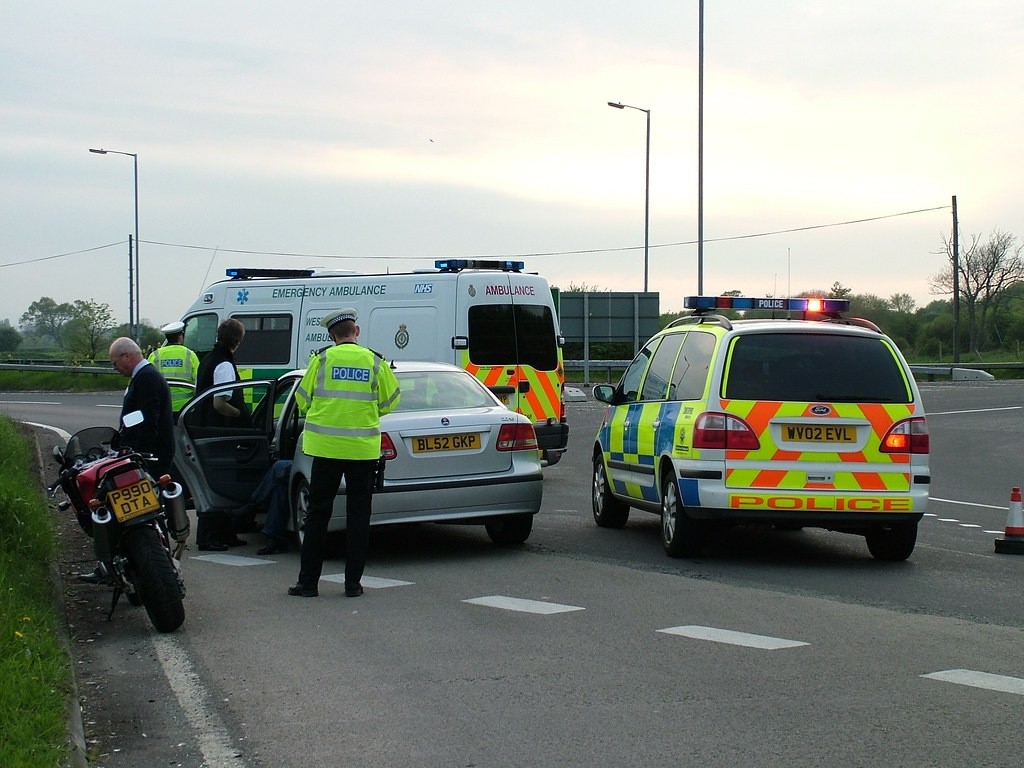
[288,582,318,597]
[346,583,363,597]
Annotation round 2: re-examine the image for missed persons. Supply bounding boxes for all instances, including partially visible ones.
[147,318,252,552]
[77,336,176,580]
[223,459,293,555]
[287,307,400,598]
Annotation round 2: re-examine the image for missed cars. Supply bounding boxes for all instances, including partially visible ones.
[171,360,544,561]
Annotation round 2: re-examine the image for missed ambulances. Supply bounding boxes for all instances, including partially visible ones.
[157,258,570,470]
[591,295,933,564]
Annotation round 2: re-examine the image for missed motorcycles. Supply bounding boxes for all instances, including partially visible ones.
[45,427,193,635]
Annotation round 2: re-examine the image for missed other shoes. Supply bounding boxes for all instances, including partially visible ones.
[257,537,288,555]
[198,540,228,551]
[78,573,99,581]
[219,537,247,547]
[225,500,261,522]
[185,499,195,509]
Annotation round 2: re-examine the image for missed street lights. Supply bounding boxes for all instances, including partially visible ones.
[608,101,650,293]
[88,148,140,349]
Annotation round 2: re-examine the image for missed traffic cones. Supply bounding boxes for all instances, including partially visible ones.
[994,485,1024,555]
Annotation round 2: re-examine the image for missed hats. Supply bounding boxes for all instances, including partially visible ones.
[320,308,358,328]
[161,322,185,334]
